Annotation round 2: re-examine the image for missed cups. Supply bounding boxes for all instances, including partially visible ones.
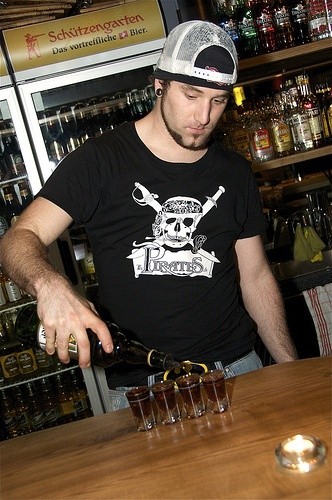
[200,370,231,413]
[150,381,179,424]
[125,386,155,430]
[176,373,207,417]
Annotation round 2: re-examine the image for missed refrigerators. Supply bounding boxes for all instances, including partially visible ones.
[0,3,183,439]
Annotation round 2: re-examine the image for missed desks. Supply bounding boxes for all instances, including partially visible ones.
[176,0,332,173]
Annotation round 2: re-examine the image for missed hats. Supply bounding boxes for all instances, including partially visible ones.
[153,46,233,92]
[157,20,237,83]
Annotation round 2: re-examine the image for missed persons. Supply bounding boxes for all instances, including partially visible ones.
[0,20,300,410]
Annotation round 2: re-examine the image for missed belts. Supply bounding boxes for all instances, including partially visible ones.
[126,348,253,388]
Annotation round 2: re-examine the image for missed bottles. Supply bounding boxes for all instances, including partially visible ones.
[14,303,176,371]
[221,74,332,162]
[0,117,34,243]
[213,0,332,60]
[38,82,157,166]
[0,264,95,441]
[80,231,97,287]
